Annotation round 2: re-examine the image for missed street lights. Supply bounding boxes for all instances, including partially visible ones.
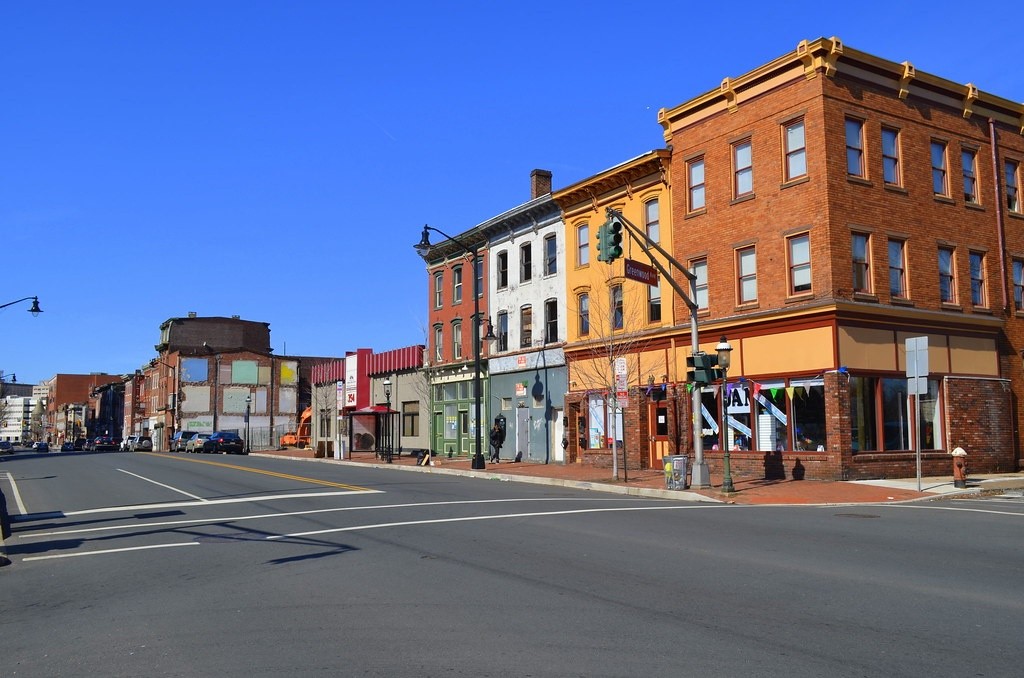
[149,358,176,440]
[383,376,394,464]
[244,395,252,452]
[715,334,735,493]
[414,224,501,469]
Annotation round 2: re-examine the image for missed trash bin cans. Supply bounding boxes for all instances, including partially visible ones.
[663,455,690,490]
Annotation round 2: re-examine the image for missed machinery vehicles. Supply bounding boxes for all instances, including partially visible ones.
[277,408,312,448]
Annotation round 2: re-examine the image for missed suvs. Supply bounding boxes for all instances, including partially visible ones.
[170,430,200,453]
[130,436,153,452]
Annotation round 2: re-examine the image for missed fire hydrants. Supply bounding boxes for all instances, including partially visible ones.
[951,445,969,489]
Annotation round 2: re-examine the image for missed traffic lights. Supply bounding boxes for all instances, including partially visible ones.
[603,221,623,262]
[596,225,603,261]
[23,419,26,427]
[686,356,707,384]
[39,421,42,427]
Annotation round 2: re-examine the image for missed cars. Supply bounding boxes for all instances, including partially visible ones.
[185,433,213,454]
[0,439,50,455]
[202,431,244,454]
[61,435,139,452]
